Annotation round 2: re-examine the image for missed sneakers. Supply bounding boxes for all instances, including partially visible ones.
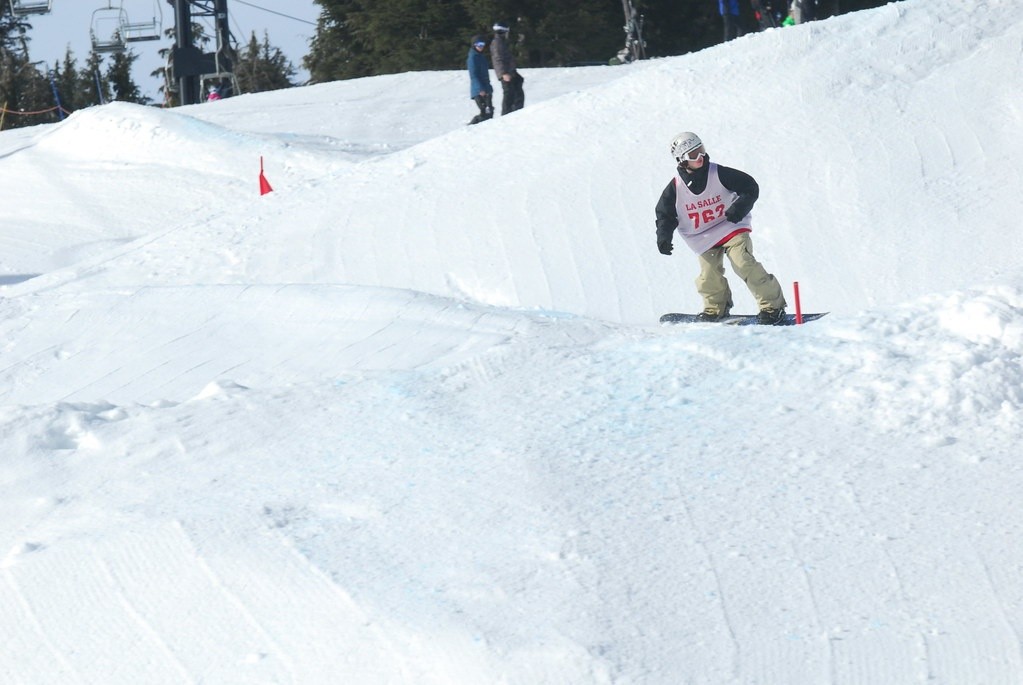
[696,307,730,322]
[758,306,786,325]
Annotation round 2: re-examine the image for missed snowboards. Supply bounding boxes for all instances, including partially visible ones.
[660,312,830,327]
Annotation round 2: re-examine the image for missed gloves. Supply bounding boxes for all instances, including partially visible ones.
[659,241,673,255]
[725,206,742,224]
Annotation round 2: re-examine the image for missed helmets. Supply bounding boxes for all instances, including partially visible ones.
[471,34,486,46]
[493,21,510,33]
[671,132,701,164]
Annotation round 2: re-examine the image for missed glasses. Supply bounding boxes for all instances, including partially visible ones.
[688,144,705,161]
[476,41,486,47]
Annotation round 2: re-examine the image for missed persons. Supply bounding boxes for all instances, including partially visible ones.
[751,0,774,30]
[207,86,222,103]
[787,0,811,25]
[467,35,493,125]
[719,0,743,42]
[654,132,788,326]
[490,21,525,115]
